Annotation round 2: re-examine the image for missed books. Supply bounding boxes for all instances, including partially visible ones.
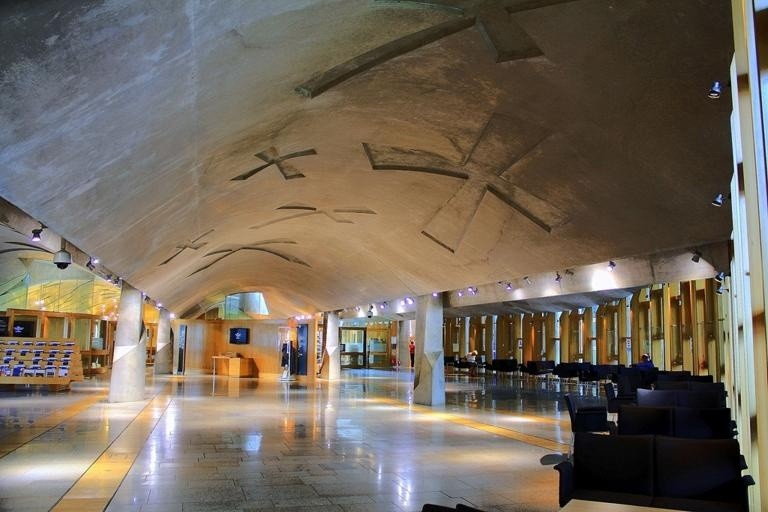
[0,340,75,376]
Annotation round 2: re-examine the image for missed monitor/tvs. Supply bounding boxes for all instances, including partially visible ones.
[230,328,248,344]
[13,320,35,337]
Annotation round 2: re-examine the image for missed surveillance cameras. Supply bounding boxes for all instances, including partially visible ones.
[54,250,71,269]
[366,311,373,318]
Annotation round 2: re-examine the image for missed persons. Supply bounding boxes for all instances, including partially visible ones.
[409,341,415,367]
[637,354,653,368]
[467,351,478,360]
[281,341,295,378]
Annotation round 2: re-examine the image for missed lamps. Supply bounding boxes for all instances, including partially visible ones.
[715,285,728,296]
[31,229,52,242]
[347,260,618,314]
[715,272,733,283]
[86,257,96,273]
[712,193,728,208]
